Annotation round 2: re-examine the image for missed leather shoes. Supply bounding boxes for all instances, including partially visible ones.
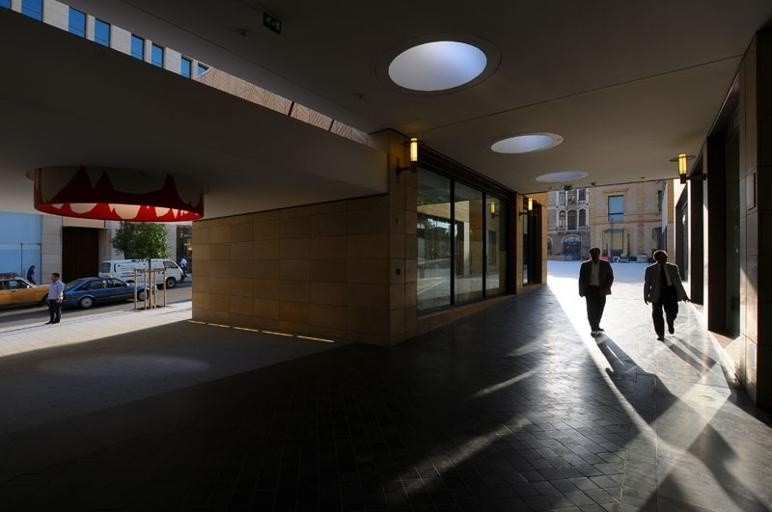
[45,321,53,324]
[52,320,60,324]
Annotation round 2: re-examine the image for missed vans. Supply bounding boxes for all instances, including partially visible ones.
[97,259,188,292]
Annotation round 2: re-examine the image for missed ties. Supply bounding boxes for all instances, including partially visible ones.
[660,264,668,291]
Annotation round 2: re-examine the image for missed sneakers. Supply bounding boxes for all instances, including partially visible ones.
[657,335,664,341]
[669,324,674,334]
[597,327,604,331]
[591,329,597,337]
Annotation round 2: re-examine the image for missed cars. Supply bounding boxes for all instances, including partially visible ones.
[0,270,51,310]
[46,273,159,309]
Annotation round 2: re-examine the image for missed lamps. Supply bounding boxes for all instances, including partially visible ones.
[518,197,536,218]
[395,137,426,184]
[669,152,696,184]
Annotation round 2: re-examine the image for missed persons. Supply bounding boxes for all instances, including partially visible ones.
[178,255,188,273]
[43,272,65,325]
[26,264,38,285]
[642,248,695,343]
[577,246,616,339]
